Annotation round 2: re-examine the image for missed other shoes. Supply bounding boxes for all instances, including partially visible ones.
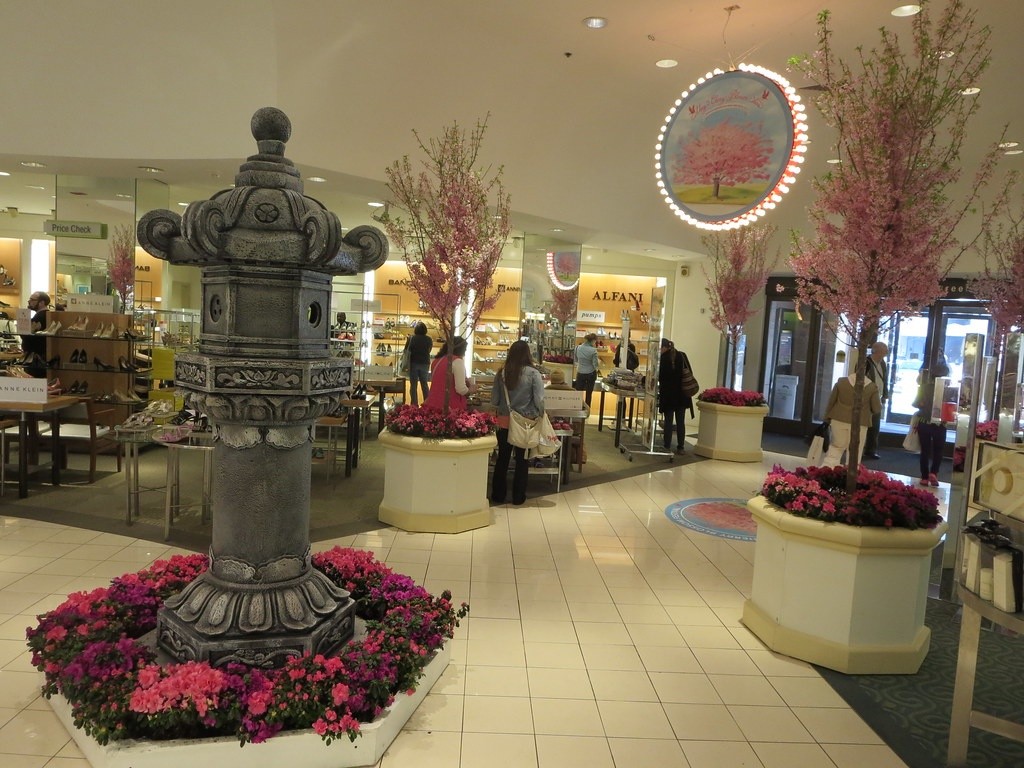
[677,448,687,454]
[929,473,939,486]
[863,453,881,461]
[660,445,671,453]
[920,479,929,485]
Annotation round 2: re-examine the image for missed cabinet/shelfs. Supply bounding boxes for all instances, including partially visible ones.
[11,308,153,455]
[371,310,648,372]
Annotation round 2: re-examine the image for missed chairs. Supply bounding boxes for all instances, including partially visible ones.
[148,348,184,423]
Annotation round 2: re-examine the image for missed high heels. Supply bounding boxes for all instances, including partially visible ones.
[619,308,650,323]
[418,297,428,308]
[593,326,621,380]
[477,320,519,377]
[1,296,209,443]
[331,310,446,357]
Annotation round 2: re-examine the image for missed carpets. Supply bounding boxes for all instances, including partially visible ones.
[811,512,1024,768]
[686,430,952,484]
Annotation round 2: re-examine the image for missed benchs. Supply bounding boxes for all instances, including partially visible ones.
[0,395,123,483]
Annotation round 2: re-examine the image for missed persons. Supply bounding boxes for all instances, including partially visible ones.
[916,365,950,486]
[658,338,693,455]
[613,328,635,426]
[819,357,881,470]
[490,339,546,504]
[331,312,356,338]
[575,332,598,408]
[919,347,949,373]
[425,336,476,411]
[862,342,888,459]
[19,291,50,378]
[403,322,432,406]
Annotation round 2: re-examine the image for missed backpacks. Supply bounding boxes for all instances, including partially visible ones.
[629,350,640,370]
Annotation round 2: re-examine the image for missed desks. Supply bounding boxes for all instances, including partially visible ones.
[597,381,646,448]
[102,410,216,542]
[0,396,83,498]
[313,378,646,491]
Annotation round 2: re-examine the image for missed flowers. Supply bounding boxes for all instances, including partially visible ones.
[953,446,967,472]
[760,460,943,531]
[684,1,1024,472]
[542,276,579,364]
[371,108,514,439]
[977,420,999,441]
[386,406,489,441]
[25,545,471,746]
[702,388,764,409]
[105,222,136,314]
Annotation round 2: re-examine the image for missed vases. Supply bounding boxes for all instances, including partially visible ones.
[739,496,948,675]
[36,641,454,768]
[542,361,573,387]
[379,427,498,533]
[695,401,769,462]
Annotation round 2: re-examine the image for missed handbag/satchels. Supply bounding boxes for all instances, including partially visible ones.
[507,409,561,459]
[902,425,921,455]
[396,350,411,379]
[805,421,828,466]
[680,350,700,397]
